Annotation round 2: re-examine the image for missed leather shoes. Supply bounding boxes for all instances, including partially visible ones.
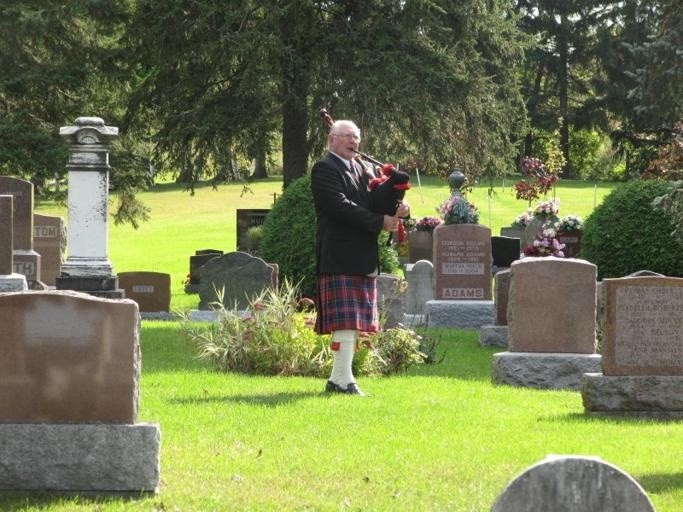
[326,380,364,396]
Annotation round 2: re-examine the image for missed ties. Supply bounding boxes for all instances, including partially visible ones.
[350,160,360,182]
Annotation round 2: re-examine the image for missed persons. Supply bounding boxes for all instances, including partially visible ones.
[311,120,410,396]
